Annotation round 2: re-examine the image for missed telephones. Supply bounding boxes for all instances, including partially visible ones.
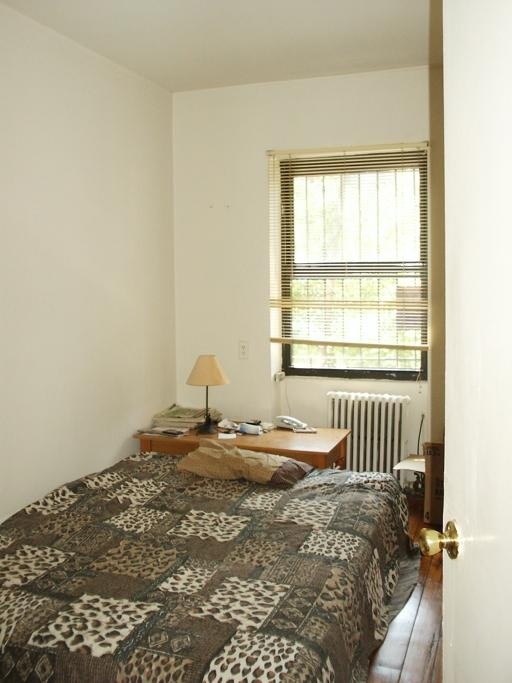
[274,415,307,429]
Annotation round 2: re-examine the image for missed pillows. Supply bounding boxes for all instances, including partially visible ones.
[176,438,314,488]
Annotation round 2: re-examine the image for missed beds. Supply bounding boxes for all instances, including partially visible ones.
[0,451,408,683]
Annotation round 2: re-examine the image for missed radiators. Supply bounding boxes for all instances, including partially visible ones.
[326,391,411,490]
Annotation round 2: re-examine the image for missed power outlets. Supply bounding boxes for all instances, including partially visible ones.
[239,342,248,358]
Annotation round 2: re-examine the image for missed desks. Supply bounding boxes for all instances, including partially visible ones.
[132,428,352,470]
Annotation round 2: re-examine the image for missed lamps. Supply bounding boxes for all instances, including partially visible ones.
[185,355,229,434]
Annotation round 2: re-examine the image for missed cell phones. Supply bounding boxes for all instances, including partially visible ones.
[162,429,183,437]
